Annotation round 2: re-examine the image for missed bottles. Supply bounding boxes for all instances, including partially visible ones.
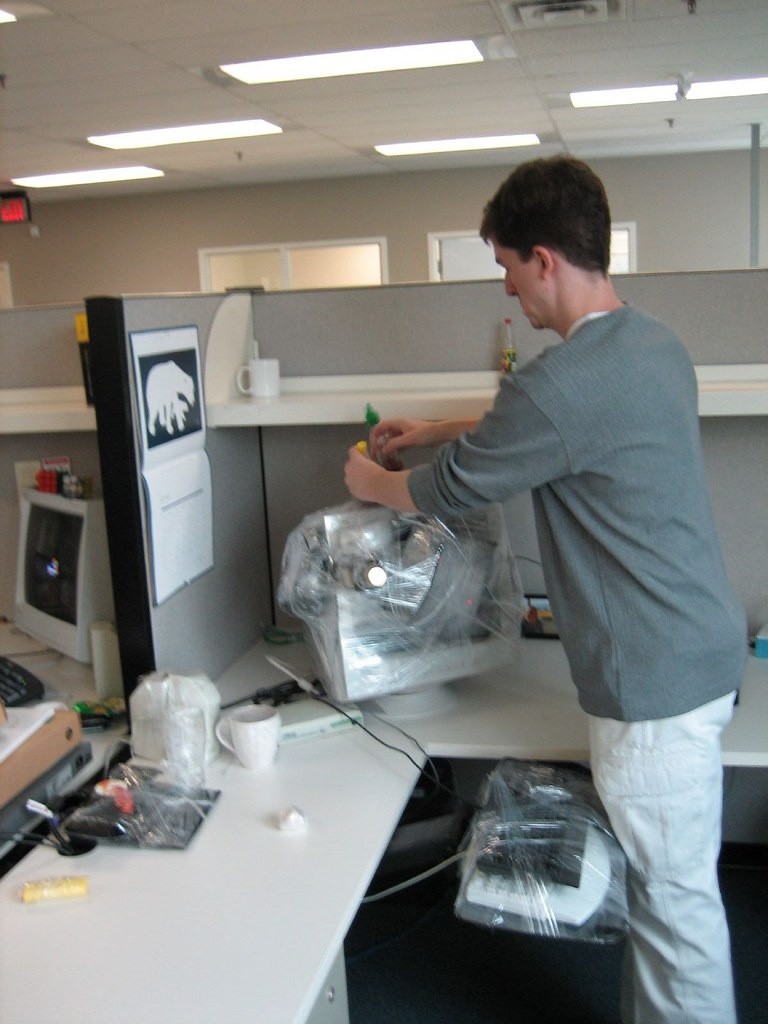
[499,318,516,376]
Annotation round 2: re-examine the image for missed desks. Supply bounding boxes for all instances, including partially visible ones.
[0,624,767,1024]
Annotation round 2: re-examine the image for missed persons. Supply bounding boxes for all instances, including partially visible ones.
[344,151,747,1024]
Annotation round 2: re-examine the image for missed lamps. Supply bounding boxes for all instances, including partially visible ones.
[374,133,542,157]
[11,166,165,188]
[219,40,485,83]
[569,77,768,108]
[84,119,283,149]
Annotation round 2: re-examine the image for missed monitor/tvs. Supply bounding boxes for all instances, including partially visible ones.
[12,484,118,662]
[301,502,518,720]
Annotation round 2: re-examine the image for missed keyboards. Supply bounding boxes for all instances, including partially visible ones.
[0,654,45,708]
[464,761,611,928]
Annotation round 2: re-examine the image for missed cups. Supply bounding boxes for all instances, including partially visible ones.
[214,704,280,768]
[237,358,280,397]
